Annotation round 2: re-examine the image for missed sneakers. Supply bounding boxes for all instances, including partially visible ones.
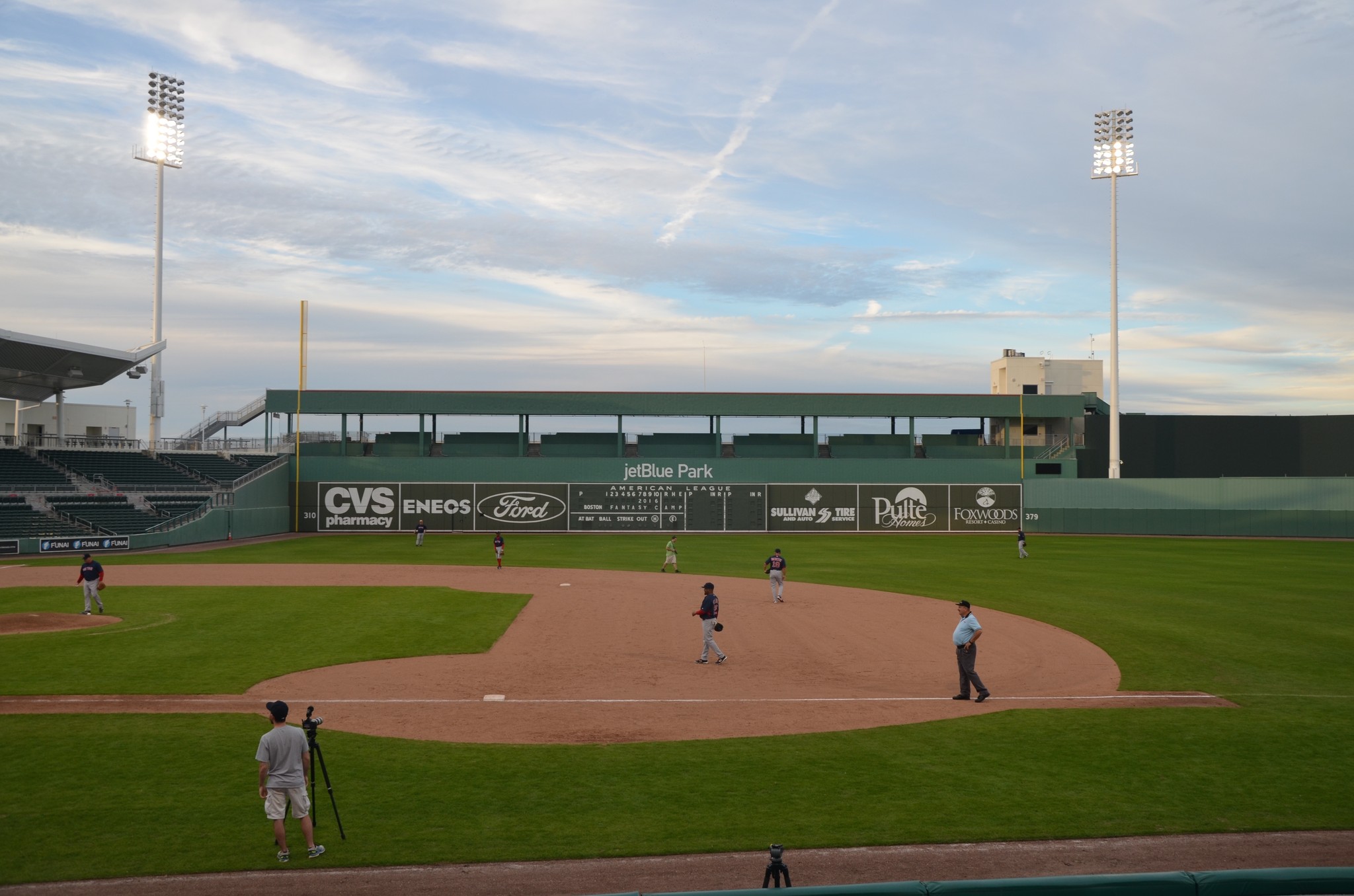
[715,655,728,664]
[776,596,784,602]
[694,659,709,664]
[307,844,325,858]
[277,849,291,863]
[773,600,777,603]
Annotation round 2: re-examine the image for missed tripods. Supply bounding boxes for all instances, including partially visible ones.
[761,843,791,888]
[274,730,346,845]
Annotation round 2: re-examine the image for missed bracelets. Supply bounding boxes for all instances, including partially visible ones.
[969,640,973,644]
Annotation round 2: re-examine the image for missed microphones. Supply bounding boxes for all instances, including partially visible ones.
[306,706,314,717]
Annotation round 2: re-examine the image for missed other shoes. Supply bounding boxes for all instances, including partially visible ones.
[420,544,422,546]
[661,568,666,572]
[496,565,502,569]
[1018,556,1023,558]
[1025,553,1029,558]
[675,569,681,573]
[80,611,91,615]
[416,545,418,546]
[99,607,104,613]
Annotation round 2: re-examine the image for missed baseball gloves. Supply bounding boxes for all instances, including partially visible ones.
[765,568,771,574]
[97,582,106,591]
[1023,542,1027,547]
[500,551,504,556]
[714,622,724,632]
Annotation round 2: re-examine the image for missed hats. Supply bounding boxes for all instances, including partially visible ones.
[955,600,970,608]
[82,553,91,559]
[266,701,289,719]
[495,531,501,534]
[1016,527,1021,530]
[701,582,714,589]
[774,549,781,554]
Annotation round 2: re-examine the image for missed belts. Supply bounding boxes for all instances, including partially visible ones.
[956,644,966,649]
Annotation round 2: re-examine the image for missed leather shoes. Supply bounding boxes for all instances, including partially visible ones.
[975,690,990,702]
[953,694,970,700]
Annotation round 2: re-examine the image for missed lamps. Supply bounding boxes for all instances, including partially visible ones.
[127,371,141,379]
[135,364,148,374]
[70,368,83,378]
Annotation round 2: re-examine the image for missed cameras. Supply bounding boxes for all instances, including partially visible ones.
[302,717,323,729]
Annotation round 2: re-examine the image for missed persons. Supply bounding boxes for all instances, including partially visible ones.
[255,699,326,862]
[661,536,682,572]
[1017,527,1028,558]
[692,583,727,665]
[953,600,990,702]
[493,531,504,569]
[76,554,106,615]
[414,520,427,547]
[764,548,786,604]
